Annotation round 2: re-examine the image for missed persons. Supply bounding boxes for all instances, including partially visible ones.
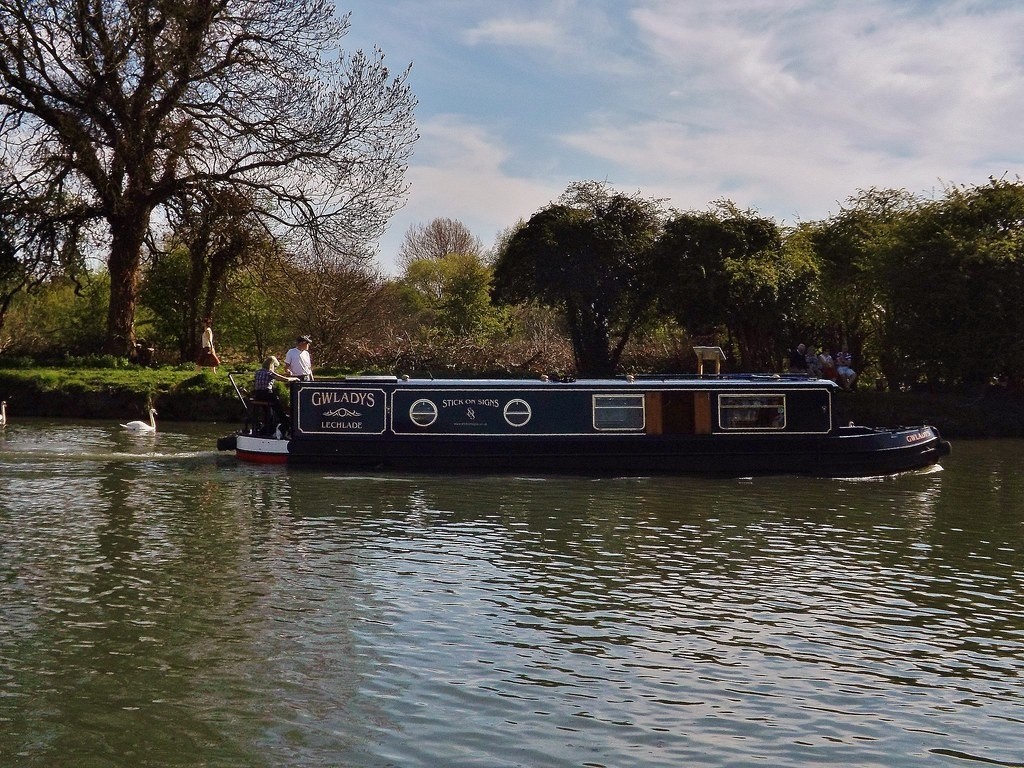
[284,335,314,381]
[788,344,857,392]
[253,356,300,400]
[198,318,220,367]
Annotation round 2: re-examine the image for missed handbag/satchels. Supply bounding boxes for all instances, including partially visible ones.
[204,353,220,366]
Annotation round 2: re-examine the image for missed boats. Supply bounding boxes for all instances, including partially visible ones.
[215,372,950,476]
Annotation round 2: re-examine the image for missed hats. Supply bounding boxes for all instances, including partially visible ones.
[300,336,312,343]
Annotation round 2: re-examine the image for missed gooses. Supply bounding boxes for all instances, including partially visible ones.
[0,401,8,425]
[119,409,158,431]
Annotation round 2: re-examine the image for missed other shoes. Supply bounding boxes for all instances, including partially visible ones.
[276,423,292,440]
